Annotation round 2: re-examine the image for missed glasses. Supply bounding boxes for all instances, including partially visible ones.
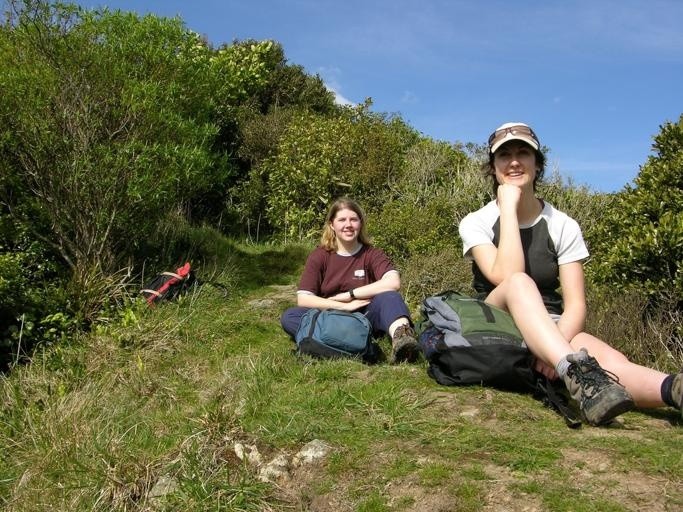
[488,125,540,145]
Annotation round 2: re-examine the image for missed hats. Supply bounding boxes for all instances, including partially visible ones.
[490,121,539,155]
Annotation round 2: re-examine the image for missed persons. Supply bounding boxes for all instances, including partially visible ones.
[457,121,682,427]
[280,198,419,364]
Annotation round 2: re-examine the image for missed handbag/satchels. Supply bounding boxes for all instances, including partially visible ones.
[296,307,372,359]
[140,261,195,309]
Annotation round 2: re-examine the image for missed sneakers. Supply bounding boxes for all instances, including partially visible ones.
[389,323,421,361]
[562,349,633,425]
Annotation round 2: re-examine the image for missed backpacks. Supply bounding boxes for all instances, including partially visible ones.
[417,284,545,389]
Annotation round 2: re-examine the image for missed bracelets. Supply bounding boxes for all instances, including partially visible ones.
[348,287,355,300]
